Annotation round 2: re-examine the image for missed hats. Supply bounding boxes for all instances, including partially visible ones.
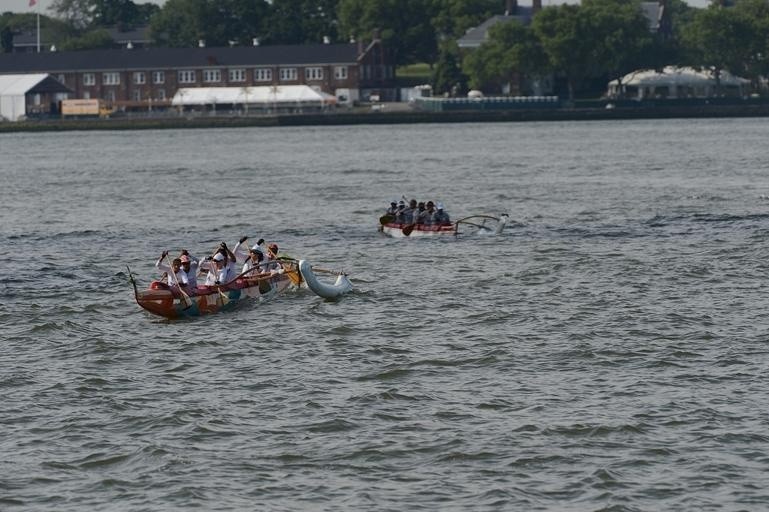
[270,243,278,249]
[212,252,226,261]
[436,201,443,210]
[391,200,405,206]
[179,254,190,263]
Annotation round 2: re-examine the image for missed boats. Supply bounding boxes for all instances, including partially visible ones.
[382,223,458,238]
[125,256,305,319]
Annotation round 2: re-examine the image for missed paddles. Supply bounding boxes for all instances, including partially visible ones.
[402,207,433,236]
[210,261,230,306]
[379,215,399,225]
[164,254,192,308]
[261,241,303,286]
[245,240,272,294]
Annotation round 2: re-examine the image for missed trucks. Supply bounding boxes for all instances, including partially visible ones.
[62,100,113,119]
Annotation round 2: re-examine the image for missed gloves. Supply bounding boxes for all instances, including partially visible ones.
[181,249,189,256]
[220,241,227,249]
[239,236,247,244]
[205,256,213,260]
[257,238,264,245]
[162,250,168,258]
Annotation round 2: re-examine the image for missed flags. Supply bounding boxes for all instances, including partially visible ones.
[29,0,38,7]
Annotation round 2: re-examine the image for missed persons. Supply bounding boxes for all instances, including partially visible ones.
[386,199,452,227]
[156,236,282,289]
